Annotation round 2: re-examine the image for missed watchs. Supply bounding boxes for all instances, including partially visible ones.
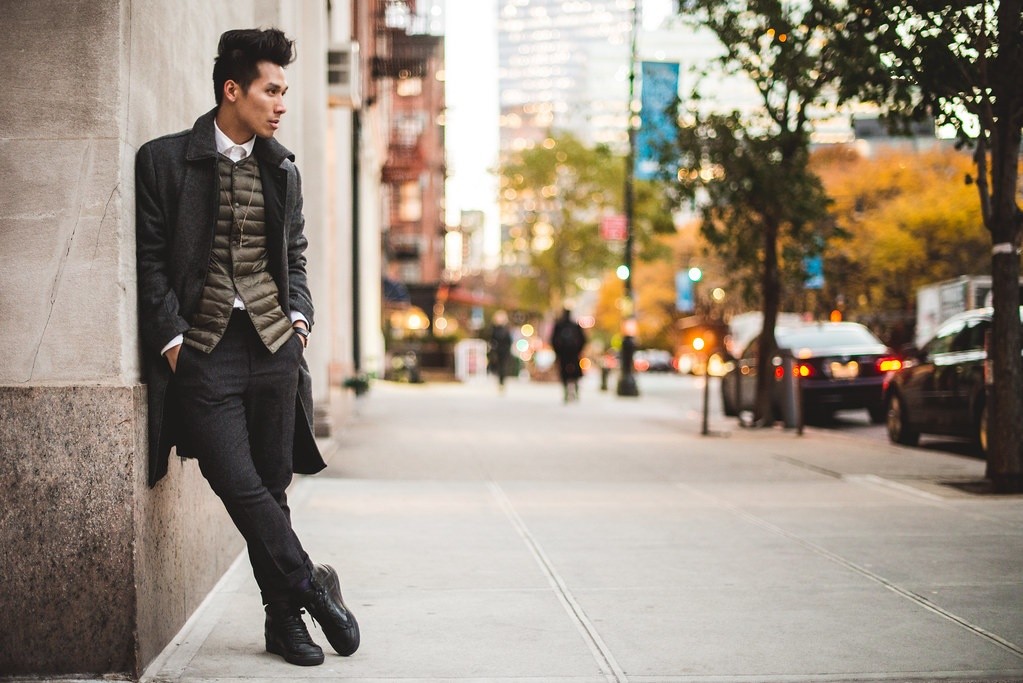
[293,327,311,348]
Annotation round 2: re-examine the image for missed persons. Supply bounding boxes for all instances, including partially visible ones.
[487,310,513,386]
[552,310,586,403]
[136,28,361,665]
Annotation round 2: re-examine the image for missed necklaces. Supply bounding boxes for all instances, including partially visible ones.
[219,166,257,248]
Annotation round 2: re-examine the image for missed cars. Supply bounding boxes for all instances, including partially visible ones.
[884,301,1023,460]
[631,348,672,372]
[719,320,904,427]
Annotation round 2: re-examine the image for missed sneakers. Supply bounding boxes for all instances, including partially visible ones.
[263,611,325,667]
[306,561,360,656]
